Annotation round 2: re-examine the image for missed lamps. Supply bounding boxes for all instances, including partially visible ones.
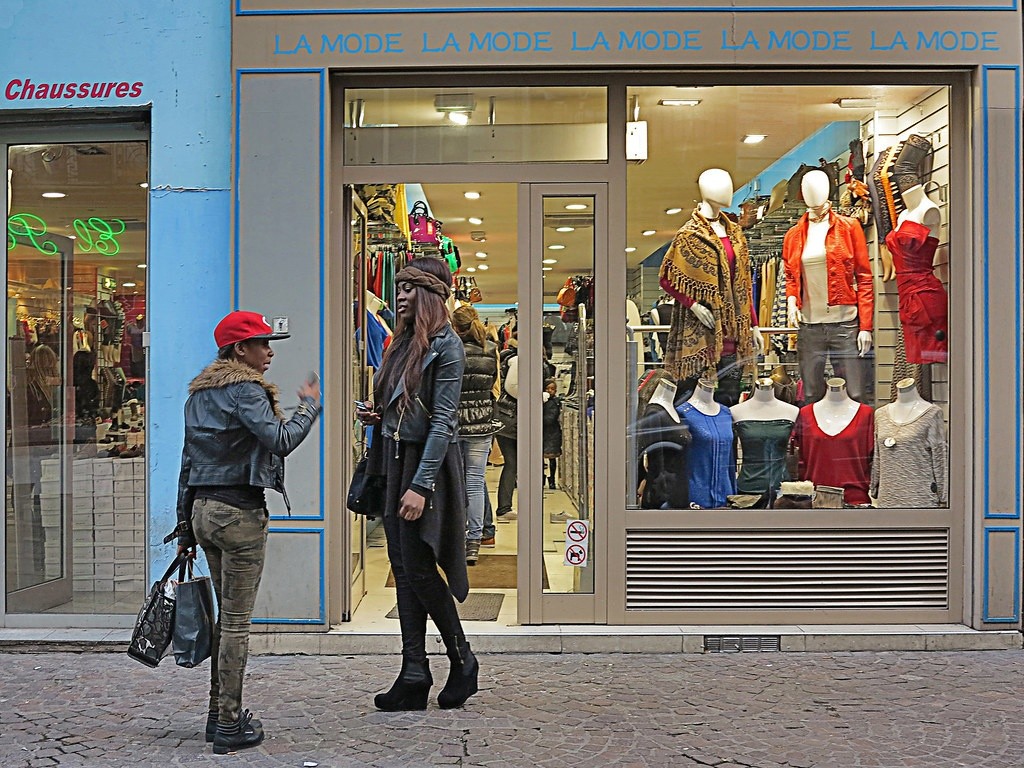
[447,111,474,127]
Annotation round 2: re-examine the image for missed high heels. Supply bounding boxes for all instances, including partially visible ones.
[374,651,434,711]
[438,641,479,708]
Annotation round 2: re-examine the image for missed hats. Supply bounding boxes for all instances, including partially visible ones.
[214,310,291,347]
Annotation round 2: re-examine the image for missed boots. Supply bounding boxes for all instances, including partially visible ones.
[774,481,814,508]
[812,486,845,510]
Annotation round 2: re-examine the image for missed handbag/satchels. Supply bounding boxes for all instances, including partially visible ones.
[452,276,482,302]
[738,157,840,229]
[557,275,593,323]
[726,493,763,509]
[408,200,461,274]
[347,424,375,515]
[126,548,215,668]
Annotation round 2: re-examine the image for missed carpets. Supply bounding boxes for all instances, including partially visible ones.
[384,554,552,589]
[385,593,506,622]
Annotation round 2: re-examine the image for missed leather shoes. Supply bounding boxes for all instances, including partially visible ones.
[213,713,265,754]
[206,708,262,741]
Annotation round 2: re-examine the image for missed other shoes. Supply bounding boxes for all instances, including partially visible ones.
[550,510,576,524]
[497,507,517,521]
[547,476,556,489]
[466,540,480,560]
[53,402,144,457]
[481,538,495,547]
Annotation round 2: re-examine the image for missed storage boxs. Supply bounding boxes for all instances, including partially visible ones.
[38,405,145,593]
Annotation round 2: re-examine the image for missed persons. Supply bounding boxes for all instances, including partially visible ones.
[782,171,874,405]
[659,169,758,407]
[498,308,518,350]
[27,344,58,425]
[638,375,948,511]
[356,257,479,711]
[885,183,947,404]
[73,299,146,415]
[176,309,324,754]
[438,306,564,567]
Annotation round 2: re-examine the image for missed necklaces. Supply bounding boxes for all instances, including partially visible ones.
[883,396,921,448]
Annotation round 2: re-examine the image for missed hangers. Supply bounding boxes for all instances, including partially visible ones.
[348,236,425,266]
[748,248,785,272]
